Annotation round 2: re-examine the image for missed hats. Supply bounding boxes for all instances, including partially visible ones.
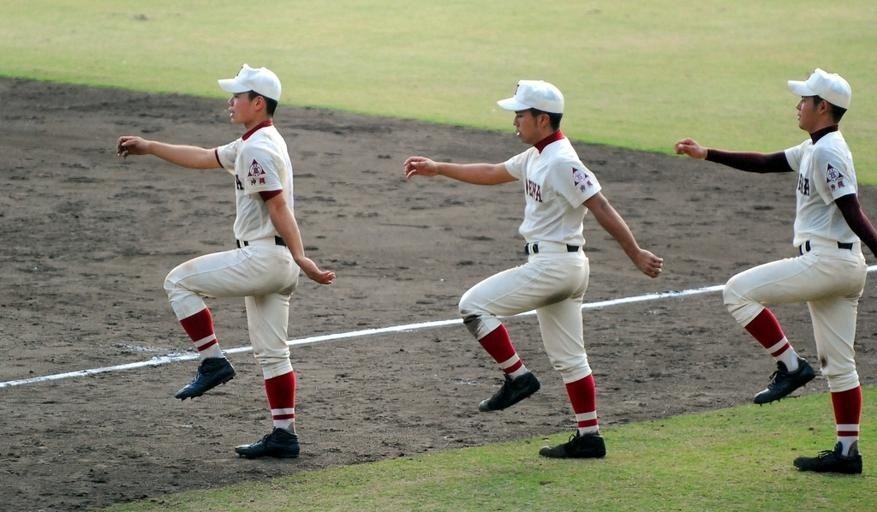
[495,78,565,115]
[215,62,282,102]
[786,66,852,111]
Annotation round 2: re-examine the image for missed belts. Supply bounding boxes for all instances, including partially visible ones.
[234,234,288,248]
[522,240,582,256]
[797,238,853,256]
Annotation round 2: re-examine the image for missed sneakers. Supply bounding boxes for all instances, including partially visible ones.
[751,353,817,407]
[477,370,541,413]
[233,425,301,460]
[792,439,863,475]
[173,355,238,401]
[537,428,607,459]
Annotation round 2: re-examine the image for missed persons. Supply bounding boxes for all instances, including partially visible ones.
[398,81,662,459]
[672,68,875,474]
[117,63,334,456]
